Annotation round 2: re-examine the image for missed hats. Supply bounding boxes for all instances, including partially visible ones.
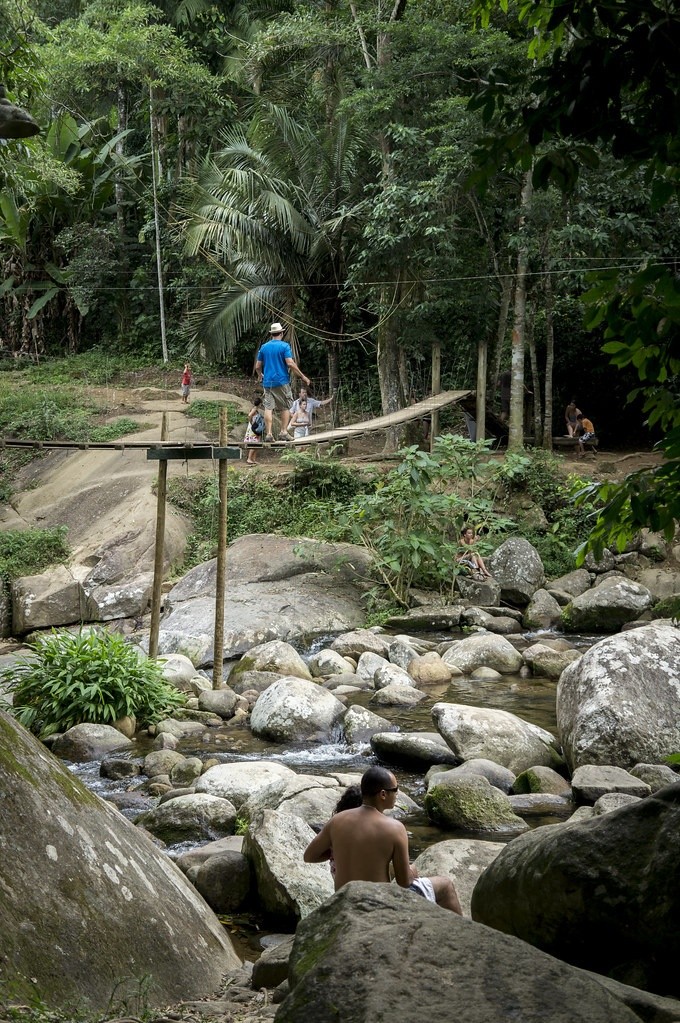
[269,323,285,333]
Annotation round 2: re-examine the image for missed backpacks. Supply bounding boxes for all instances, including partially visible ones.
[251,409,266,436]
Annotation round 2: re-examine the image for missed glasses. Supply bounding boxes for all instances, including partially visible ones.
[384,785,399,793]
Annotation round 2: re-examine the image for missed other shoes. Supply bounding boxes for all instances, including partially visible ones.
[280,432,295,441]
[265,434,275,441]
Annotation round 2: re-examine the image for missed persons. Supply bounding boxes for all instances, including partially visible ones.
[499,363,534,422]
[577,414,598,459]
[563,400,582,439]
[329,784,363,880]
[181,363,191,404]
[303,767,463,918]
[255,323,311,442]
[291,397,312,453]
[290,387,334,417]
[455,528,492,580]
[243,397,263,464]
